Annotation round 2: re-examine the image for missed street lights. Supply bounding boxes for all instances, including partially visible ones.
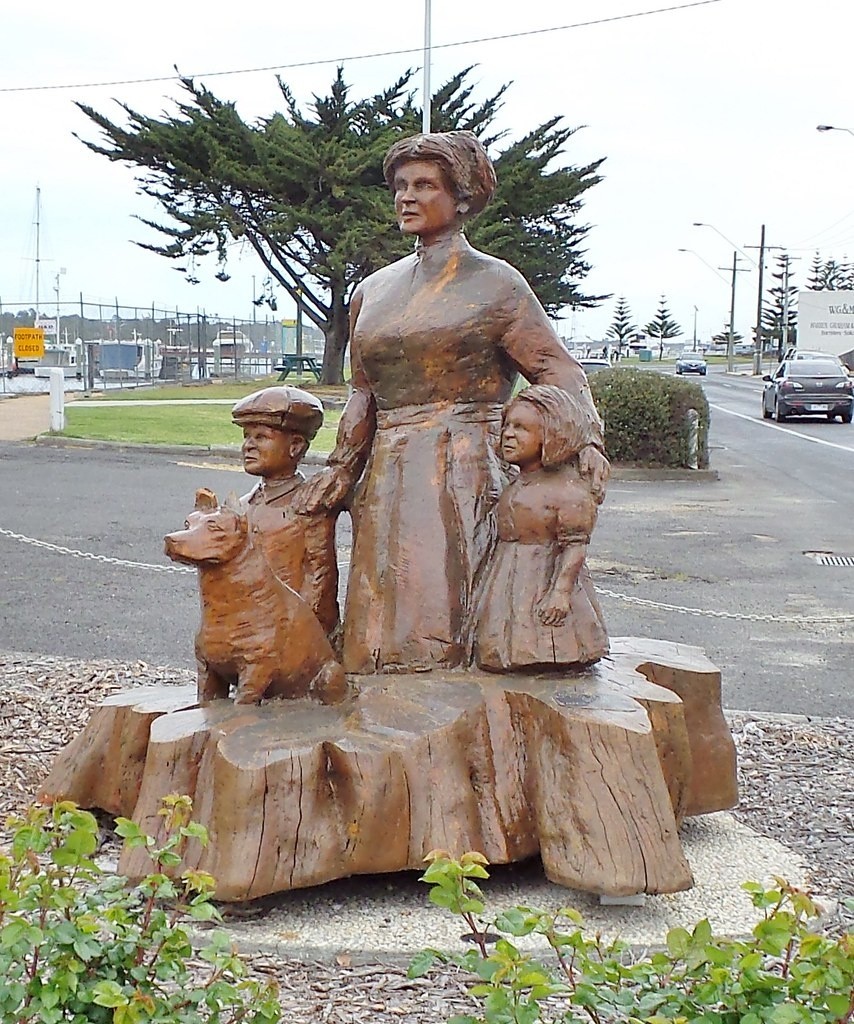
[691,219,792,357]
[677,246,735,372]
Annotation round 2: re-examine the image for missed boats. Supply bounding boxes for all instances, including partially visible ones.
[196,330,259,367]
[98,339,163,379]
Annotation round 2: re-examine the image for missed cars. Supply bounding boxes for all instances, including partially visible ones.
[760,358,854,423]
[676,351,707,375]
[782,347,850,376]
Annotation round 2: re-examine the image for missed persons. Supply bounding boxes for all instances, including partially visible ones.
[291,128,611,673]
[477,382,612,669]
[228,385,341,637]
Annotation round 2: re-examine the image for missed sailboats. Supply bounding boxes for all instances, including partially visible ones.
[7,186,88,378]
